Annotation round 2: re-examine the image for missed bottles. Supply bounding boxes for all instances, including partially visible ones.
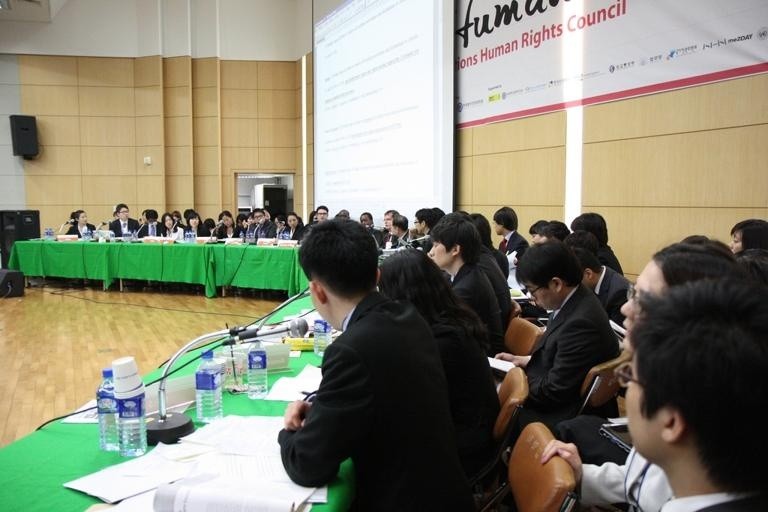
[43,227,291,246]
[97,317,333,456]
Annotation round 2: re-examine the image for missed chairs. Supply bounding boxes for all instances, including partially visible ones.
[492,301,632,511]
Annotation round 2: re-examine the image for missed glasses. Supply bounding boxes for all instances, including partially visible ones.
[119,210,129,213]
[254,215,262,219]
[612,362,643,388]
[625,284,639,302]
[317,213,326,215]
[527,286,540,299]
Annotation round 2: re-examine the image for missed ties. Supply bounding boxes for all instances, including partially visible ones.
[255,227,259,238]
[500,239,507,253]
[151,225,154,236]
[123,223,126,228]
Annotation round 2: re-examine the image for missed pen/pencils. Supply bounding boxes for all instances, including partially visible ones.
[301,391,310,396]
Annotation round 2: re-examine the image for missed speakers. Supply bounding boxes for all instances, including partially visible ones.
[0,269,24,297]
[0,210,41,269]
[9,114,39,156]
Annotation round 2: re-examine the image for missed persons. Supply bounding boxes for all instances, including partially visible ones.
[64,204,290,240]
[276,204,768,511]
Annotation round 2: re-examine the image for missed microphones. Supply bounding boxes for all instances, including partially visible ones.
[257,215,266,226]
[102,219,112,224]
[222,317,309,342]
[216,220,224,228]
[277,221,285,231]
[66,218,75,224]
[175,217,180,223]
[409,233,431,243]
